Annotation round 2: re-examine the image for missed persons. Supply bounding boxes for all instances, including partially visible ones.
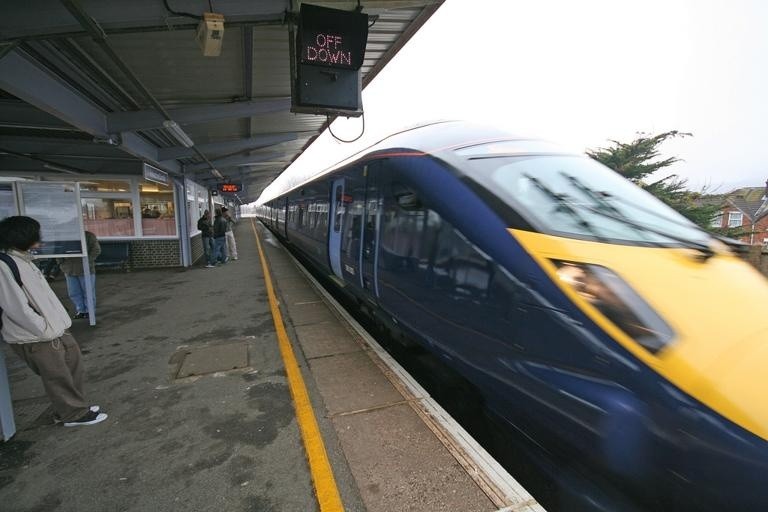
[142,209,154,218]
[220,207,238,260]
[56,231,101,319]
[0,216,108,426]
[205,209,227,267]
[151,206,162,218]
[197,210,222,262]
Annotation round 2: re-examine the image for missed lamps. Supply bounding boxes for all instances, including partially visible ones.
[163,119,194,149]
[211,168,222,179]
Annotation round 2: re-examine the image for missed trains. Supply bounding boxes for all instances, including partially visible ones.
[256,116,768,511]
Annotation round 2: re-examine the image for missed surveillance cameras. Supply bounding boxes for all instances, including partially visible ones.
[194,12,225,57]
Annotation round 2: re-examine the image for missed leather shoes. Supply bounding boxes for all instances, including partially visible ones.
[73,313,89,318]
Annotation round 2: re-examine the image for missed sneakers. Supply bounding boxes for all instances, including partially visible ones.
[64,412,108,427]
[52,406,99,423]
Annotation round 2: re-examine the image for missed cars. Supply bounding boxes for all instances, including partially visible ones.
[88,205,161,220]
